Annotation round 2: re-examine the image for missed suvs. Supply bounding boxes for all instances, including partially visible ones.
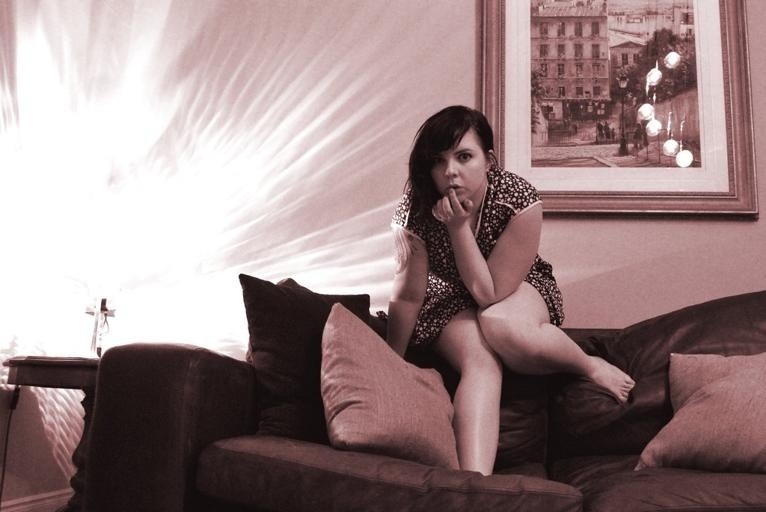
[548,119,577,140]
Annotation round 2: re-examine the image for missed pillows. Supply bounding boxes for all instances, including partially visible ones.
[632,350,765,470]
[238,272,374,437]
[317,300,462,468]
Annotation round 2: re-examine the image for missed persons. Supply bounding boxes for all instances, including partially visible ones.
[386,105,636,476]
[598,121,616,140]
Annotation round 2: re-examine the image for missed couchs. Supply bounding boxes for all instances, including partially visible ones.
[72,327,765,511]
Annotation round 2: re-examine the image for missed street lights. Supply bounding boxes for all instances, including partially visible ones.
[616,73,630,157]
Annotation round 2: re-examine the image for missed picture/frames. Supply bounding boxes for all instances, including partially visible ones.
[479,0,760,219]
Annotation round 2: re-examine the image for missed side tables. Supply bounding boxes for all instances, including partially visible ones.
[0,354,99,512]
